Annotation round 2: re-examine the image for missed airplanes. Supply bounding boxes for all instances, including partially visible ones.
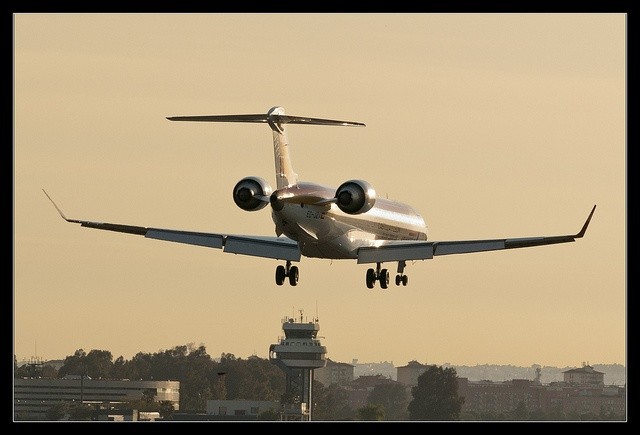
[42,106,596,290]
